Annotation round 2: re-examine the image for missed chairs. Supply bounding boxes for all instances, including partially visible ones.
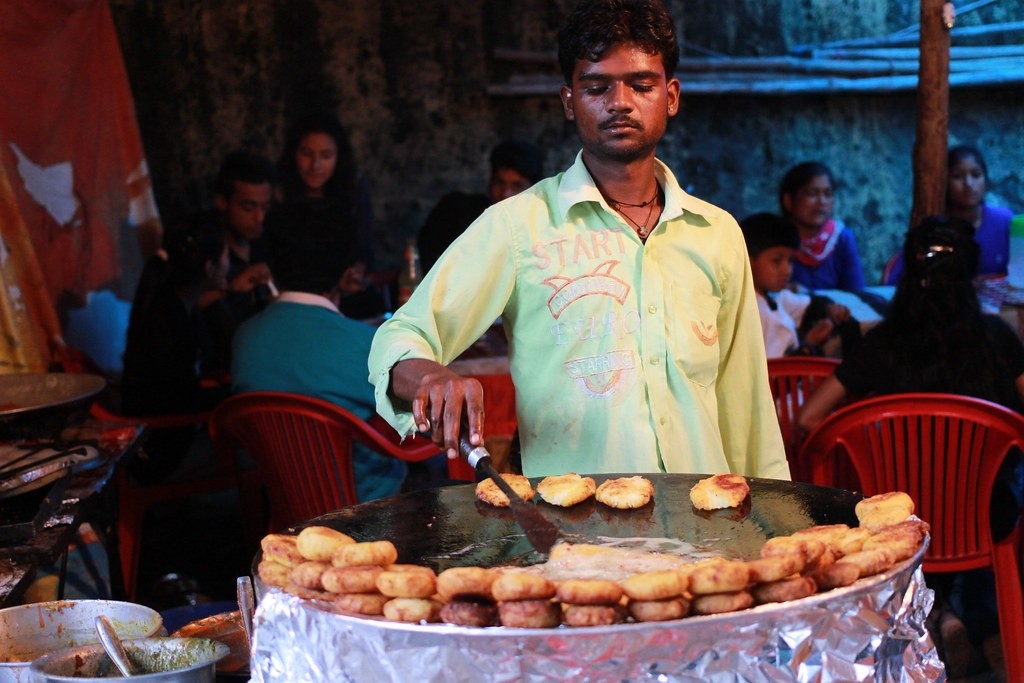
[218,385,443,536]
[767,356,843,483]
[90,376,260,603]
[798,392,1024,683]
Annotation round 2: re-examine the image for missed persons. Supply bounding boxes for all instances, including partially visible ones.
[367,0,792,482]
[417,140,544,271]
[231,209,454,503]
[123,213,255,476]
[799,214,1024,678]
[778,161,866,291]
[741,213,847,418]
[199,112,387,340]
[888,144,1013,286]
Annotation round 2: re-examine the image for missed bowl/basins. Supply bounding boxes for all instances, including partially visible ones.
[30,637,231,683]
[0,599,164,682]
[0,373,107,437]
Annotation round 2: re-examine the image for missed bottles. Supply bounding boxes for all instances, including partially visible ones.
[400,237,421,309]
[1008,216,1024,289]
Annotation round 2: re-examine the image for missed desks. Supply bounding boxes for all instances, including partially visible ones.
[0,418,148,612]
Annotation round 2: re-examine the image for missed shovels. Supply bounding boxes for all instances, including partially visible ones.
[458,430,573,553]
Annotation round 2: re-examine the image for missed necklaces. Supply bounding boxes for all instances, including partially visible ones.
[604,178,660,236]
